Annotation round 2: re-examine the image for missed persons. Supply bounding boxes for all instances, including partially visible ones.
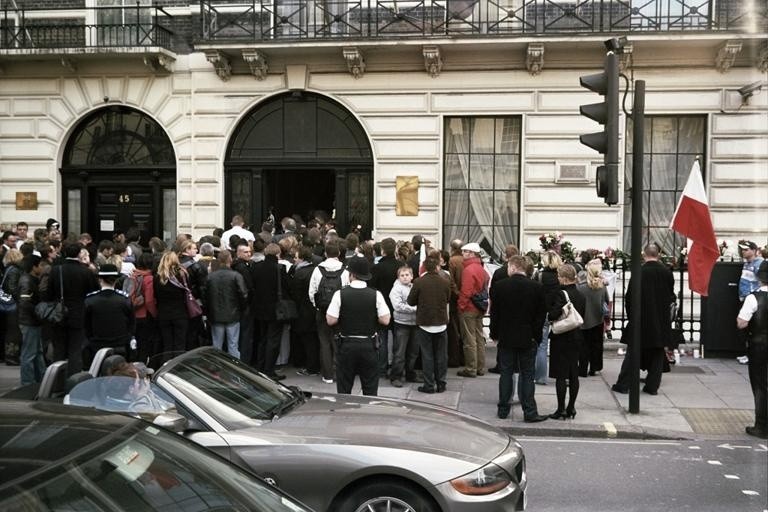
[756,246,768,259]
[615,243,673,395]
[105,363,146,422]
[737,241,762,364]
[736,260,768,438]
[661,256,677,365]
[129,360,178,414]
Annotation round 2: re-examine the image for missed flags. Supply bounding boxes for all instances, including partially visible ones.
[673,161,719,298]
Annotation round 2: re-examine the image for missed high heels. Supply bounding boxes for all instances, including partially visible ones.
[548,408,576,420]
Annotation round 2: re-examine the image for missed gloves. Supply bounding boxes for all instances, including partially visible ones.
[129,338,139,350]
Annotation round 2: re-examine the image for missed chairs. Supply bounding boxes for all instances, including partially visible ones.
[100,354,127,380]
[63,370,96,407]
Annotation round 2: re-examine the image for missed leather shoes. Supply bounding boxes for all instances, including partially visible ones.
[744,425,768,439]
[6,357,20,367]
[524,414,548,424]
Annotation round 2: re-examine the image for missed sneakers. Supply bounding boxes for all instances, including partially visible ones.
[498,404,511,419]
[612,383,630,395]
[297,368,334,385]
[579,366,597,378]
[391,375,447,392]
[737,354,750,364]
[458,367,500,377]
[272,363,287,381]
[643,386,658,394]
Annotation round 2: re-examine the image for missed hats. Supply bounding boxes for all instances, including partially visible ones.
[461,242,480,252]
[132,362,156,378]
[754,260,768,282]
[738,240,758,250]
[97,264,122,278]
[348,256,373,280]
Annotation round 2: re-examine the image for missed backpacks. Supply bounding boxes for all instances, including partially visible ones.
[315,263,345,308]
[123,271,147,308]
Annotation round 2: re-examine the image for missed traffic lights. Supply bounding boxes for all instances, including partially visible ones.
[593,162,617,205]
[578,55,621,151]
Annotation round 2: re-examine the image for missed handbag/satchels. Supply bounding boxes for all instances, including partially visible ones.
[34,301,68,326]
[0,287,18,313]
[469,287,489,313]
[547,290,583,334]
[185,287,203,318]
[276,300,299,320]
